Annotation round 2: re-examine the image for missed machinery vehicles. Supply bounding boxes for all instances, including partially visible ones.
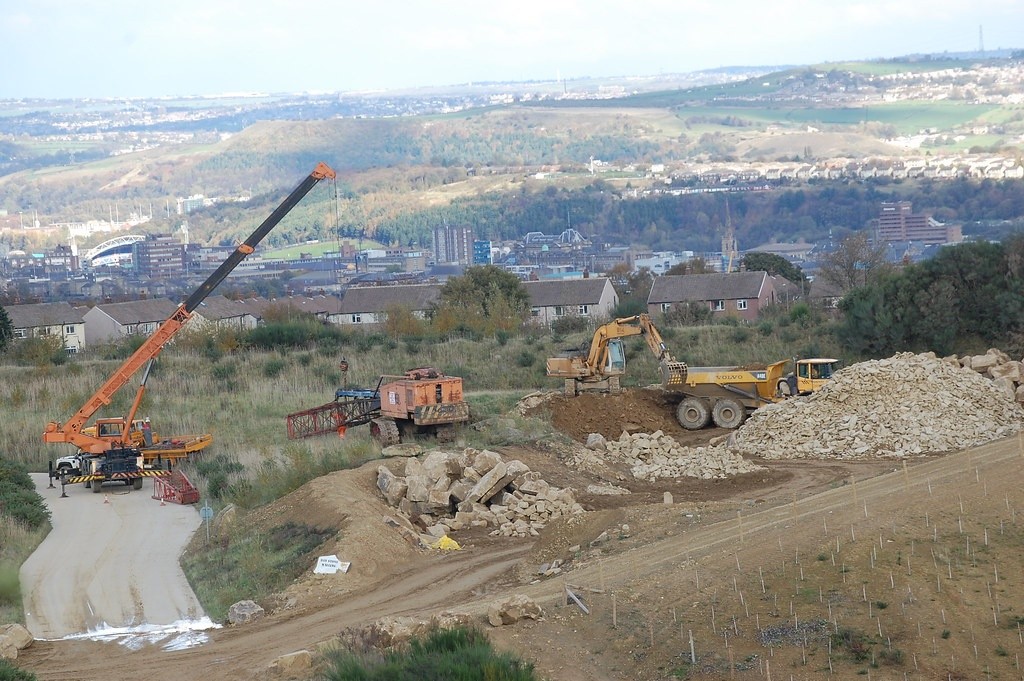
[371,367,470,449]
[546,313,687,397]
[44,161,336,494]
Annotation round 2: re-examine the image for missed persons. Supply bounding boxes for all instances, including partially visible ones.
[101,425,109,434]
[812,368,820,379]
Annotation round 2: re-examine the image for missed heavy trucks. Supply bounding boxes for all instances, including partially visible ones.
[671,354,843,430]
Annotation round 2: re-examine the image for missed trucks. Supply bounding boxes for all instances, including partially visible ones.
[56,429,211,478]
